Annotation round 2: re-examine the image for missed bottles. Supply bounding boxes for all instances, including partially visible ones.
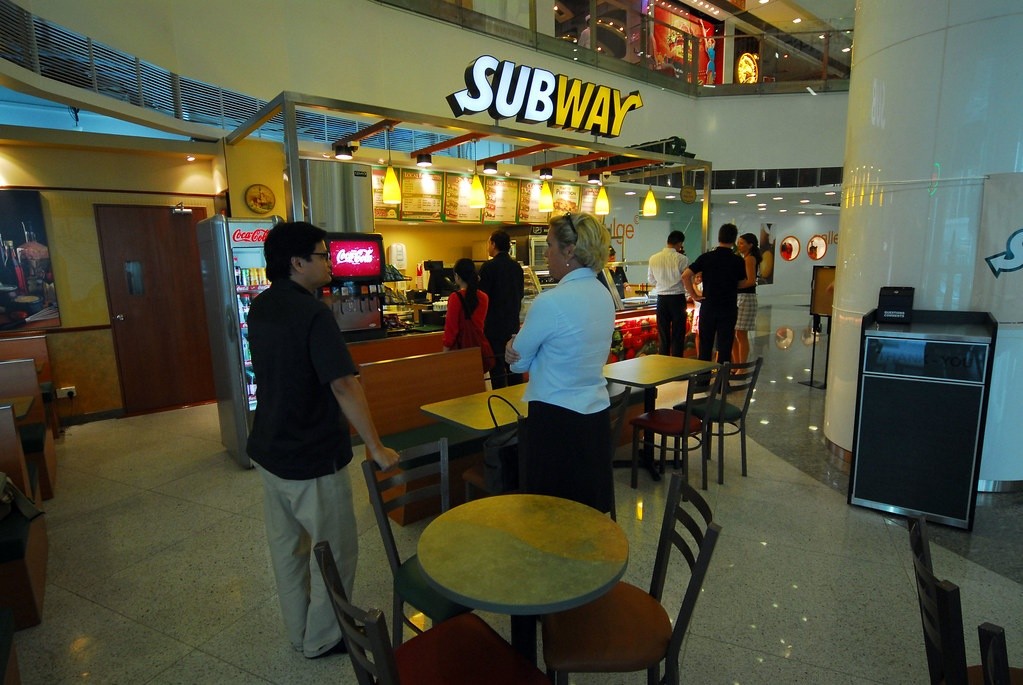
[0,234,26,295]
[233,257,242,286]
[433,300,448,311]
[16,220,50,296]
[237,294,259,323]
[399,300,415,327]
[242,333,251,361]
[246,367,257,400]
[250,268,271,285]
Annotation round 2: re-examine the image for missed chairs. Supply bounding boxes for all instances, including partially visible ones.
[629,366,724,502]
[361,437,475,648]
[540,471,722,685]
[462,416,528,503]
[603,354,721,482]
[313,541,551,685]
[671,355,763,484]
[610,386,630,458]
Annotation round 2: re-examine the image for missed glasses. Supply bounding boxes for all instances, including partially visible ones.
[302,251,331,262]
[563,212,577,234]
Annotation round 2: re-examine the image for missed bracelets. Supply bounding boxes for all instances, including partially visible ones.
[625,285,630,288]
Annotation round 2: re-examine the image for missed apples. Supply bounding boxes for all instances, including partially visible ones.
[621,320,659,359]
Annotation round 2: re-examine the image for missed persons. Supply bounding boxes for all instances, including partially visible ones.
[728,233,764,375]
[474,229,524,390]
[505,211,616,512]
[681,223,747,393]
[595,243,633,311]
[577,14,591,49]
[648,229,690,359]
[245,223,401,658]
[442,258,496,387]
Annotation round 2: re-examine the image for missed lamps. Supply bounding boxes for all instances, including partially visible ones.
[483,161,498,175]
[382,128,402,205]
[642,160,658,216]
[538,151,554,212]
[468,141,487,209]
[416,154,433,167]
[539,167,553,181]
[594,173,609,215]
[334,145,353,161]
[588,173,600,184]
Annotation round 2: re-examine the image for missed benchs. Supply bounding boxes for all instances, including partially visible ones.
[0,404,48,630]
[0,359,57,502]
[0,335,60,438]
[359,344,486,526]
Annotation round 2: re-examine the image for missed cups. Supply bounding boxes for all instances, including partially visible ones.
[0,284,18,305]
[13,294,44,316]
[417,264,423,290]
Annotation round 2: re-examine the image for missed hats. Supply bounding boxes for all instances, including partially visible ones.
[608,245,616,254]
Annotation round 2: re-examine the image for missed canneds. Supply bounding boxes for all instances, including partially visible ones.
[241,267,269,286]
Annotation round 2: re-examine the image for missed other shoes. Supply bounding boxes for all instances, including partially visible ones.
[306,626,366,659]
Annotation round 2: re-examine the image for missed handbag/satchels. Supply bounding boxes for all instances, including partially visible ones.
[447,291,495,373]
[482,394,526,493]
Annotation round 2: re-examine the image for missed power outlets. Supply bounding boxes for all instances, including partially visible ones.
[56,386,77,399]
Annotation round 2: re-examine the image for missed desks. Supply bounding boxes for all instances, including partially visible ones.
[418,382,528,498]
[0,397,35,420]
[416,494,629,665]
